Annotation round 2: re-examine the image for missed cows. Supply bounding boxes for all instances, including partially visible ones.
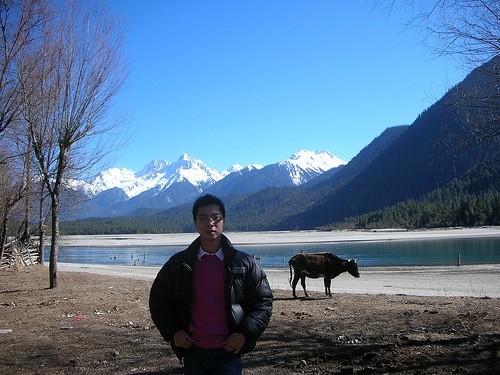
[289,252,361,299]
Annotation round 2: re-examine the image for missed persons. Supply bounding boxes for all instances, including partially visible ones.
[148,193,274,375]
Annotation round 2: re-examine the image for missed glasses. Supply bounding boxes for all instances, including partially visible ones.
[192,214,224,224]
